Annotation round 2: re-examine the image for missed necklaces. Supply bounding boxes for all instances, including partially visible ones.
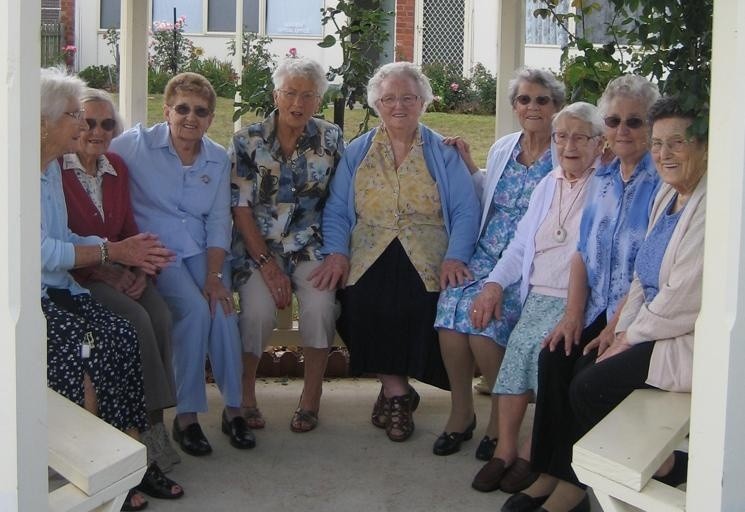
[553,171,593,242]
[619,165,638,183]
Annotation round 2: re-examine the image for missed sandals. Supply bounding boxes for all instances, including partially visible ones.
[387,388,421,443]
[290,394,323,435]
[122,488,149,512]
[371,383,392,427]
[244,405,265,428]
[139,462,183,499]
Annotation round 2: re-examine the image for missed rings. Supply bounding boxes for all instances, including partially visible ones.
[278,288,281,292]
[223,296,231,304]
[472,309,479,313]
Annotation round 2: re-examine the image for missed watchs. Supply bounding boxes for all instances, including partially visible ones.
[209,271,225,279]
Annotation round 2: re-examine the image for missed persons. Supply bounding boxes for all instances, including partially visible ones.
[569,91,710,487]
[228,58,346,432]
[501,75,664,512]
[40,65,184,511]
[107,73,256,456]
[470,102,607,493]
[306,61,480,442]
[433,67,566,460]
[56,88,180,472]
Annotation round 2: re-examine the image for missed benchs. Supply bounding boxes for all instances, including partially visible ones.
[48,289,690,512]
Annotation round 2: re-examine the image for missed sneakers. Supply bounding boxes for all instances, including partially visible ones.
[151,423,183,464]
[139,432,172,473]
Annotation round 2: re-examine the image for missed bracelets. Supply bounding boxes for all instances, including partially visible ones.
[99,241,108,265]
[254,248,274,270]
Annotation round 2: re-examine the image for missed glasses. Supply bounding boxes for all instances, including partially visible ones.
[70,109,86,121]
[648,139,686,153]
[604,117,643,128]
[84,118,116,131]
[283,90,315,98]
[174,104,210,118]
[381,94,418,105]
[553,132,590,145]
[516,94,551,105]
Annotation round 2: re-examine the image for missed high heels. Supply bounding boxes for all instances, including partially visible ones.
[475,434,501,460]
[432,412,477,455]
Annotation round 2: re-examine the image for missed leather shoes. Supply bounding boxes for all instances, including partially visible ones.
[536,492,593,511]
[498,456,541,493]
[220,411,257,450]
[472,456,505,491]
[171,417,212,457]
[500,492,550,511]
